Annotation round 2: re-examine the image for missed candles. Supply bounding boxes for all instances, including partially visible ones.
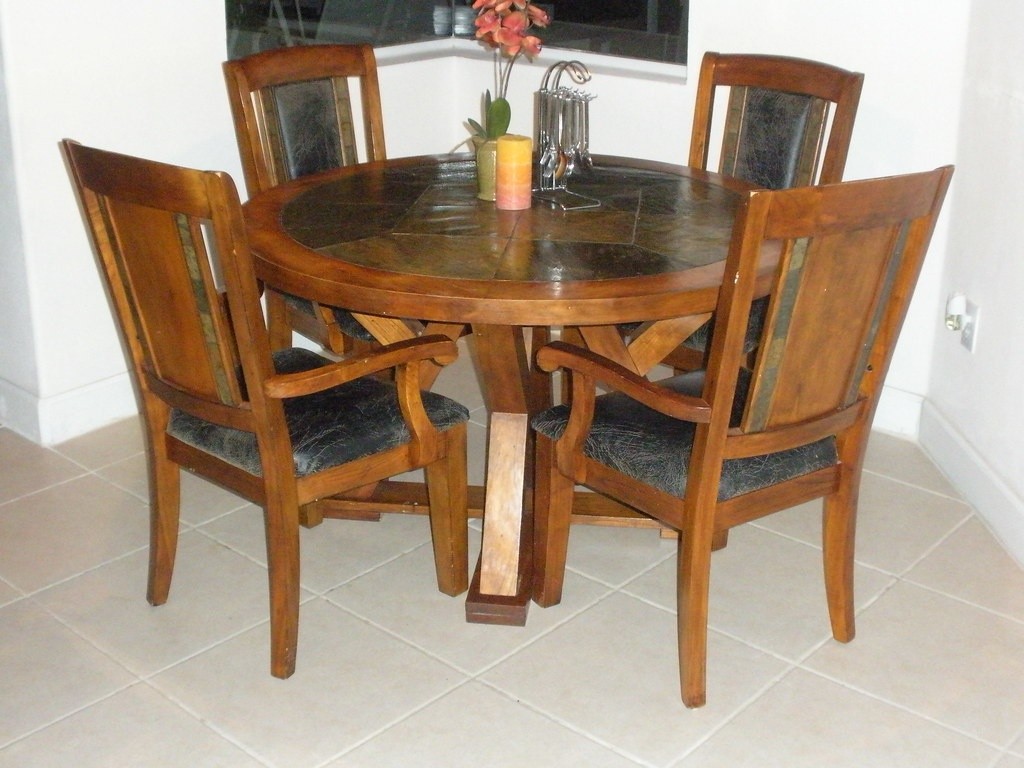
[495,135,533,209]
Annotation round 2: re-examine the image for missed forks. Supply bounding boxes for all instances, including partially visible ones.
[539,89,593,179]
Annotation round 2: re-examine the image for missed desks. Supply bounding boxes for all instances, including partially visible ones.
[237,154,808,626]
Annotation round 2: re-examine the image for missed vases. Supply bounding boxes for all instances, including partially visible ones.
[475,134,513,202]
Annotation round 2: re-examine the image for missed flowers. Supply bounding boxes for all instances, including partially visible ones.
[466,0,552,137]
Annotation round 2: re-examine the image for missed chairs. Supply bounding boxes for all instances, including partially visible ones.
[560,51,864,406]
[219,42,475,379]
[62,139,469,676]
[530,164,953,709]
